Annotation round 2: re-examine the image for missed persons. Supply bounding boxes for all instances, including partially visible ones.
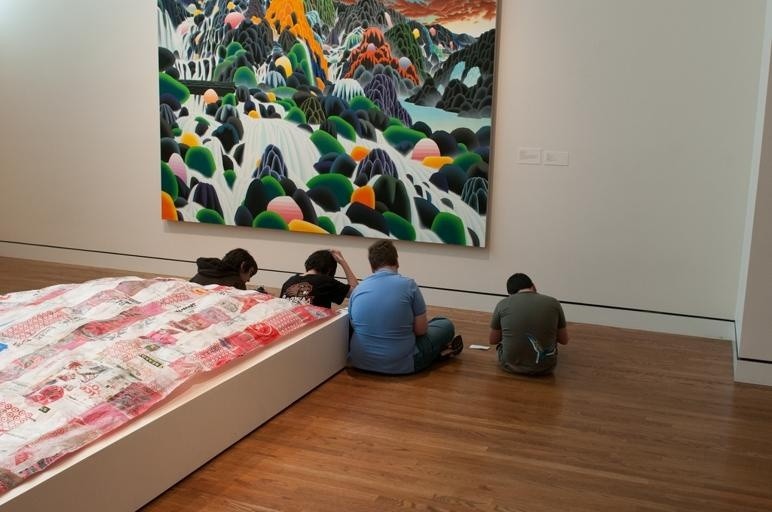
[188,248,269,296]
[278,248,359,311]
[487,272,569,376]
[345,238,463,376]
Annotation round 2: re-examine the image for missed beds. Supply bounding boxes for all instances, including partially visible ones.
[0,276,350,512]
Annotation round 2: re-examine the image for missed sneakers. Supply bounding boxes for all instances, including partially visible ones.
[437,335,463,363]
